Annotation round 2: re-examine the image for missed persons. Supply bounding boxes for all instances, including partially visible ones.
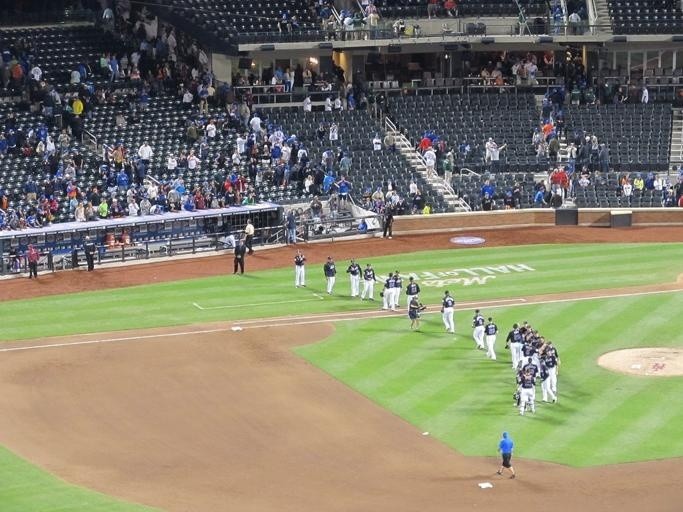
[405,276,423,329]
[472,310,486,349]
[381,270,402,311]
[498,433,515,479]
[0,1,682,280]
[346,259,363,297]
[324,256,336,294]
[361,264,376,301]
[294,248,306,288]
[506,322,560,416]
[484,318,497,360]
[441,290,456,333]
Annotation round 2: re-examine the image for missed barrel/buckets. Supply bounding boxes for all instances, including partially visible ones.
[466,22,487,35]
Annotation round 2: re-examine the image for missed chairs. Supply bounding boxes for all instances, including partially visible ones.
[377,0,682,217]
[0,0,375,274]
